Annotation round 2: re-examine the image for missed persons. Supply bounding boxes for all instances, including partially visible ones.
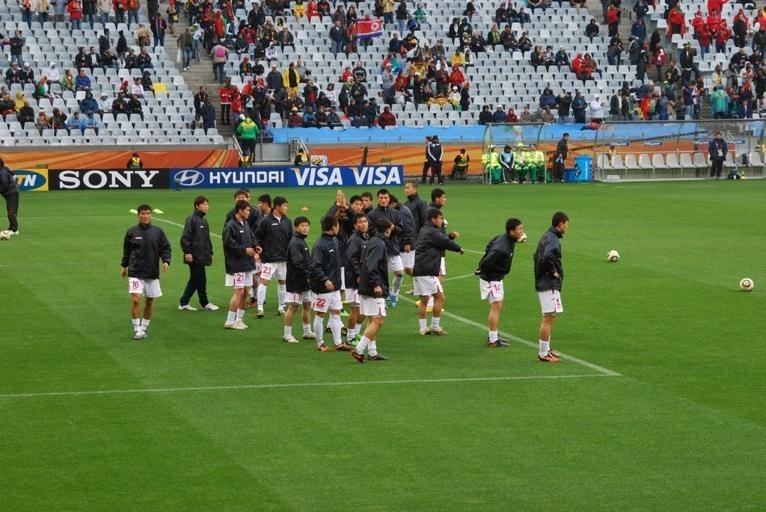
[0,158,20,239]
[0,0,765,188]
[532,210,571,363]
[176,195,221,313]
[472,217,524,348]
[119,202,173,341]
[221,177,466,366]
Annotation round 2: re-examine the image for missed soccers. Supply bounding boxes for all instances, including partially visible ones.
[739,277,754,291]
[0,230,10,240]
[607,249,620,262]
[518,233,527,242]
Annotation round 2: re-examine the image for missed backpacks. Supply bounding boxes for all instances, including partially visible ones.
[216,47,225,57]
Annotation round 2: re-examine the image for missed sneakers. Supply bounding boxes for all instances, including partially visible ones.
[183,65,190,71]
[421,179,564,185]
[7,229,20,234]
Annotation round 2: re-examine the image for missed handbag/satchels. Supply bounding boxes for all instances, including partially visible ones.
[176,48,182,63]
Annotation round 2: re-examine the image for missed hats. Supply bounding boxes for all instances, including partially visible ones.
[516,143,526,148]
[231,64,502,120]
[487,144,495,149]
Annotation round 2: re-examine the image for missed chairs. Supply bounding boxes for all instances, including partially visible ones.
[595,148,762,183]
